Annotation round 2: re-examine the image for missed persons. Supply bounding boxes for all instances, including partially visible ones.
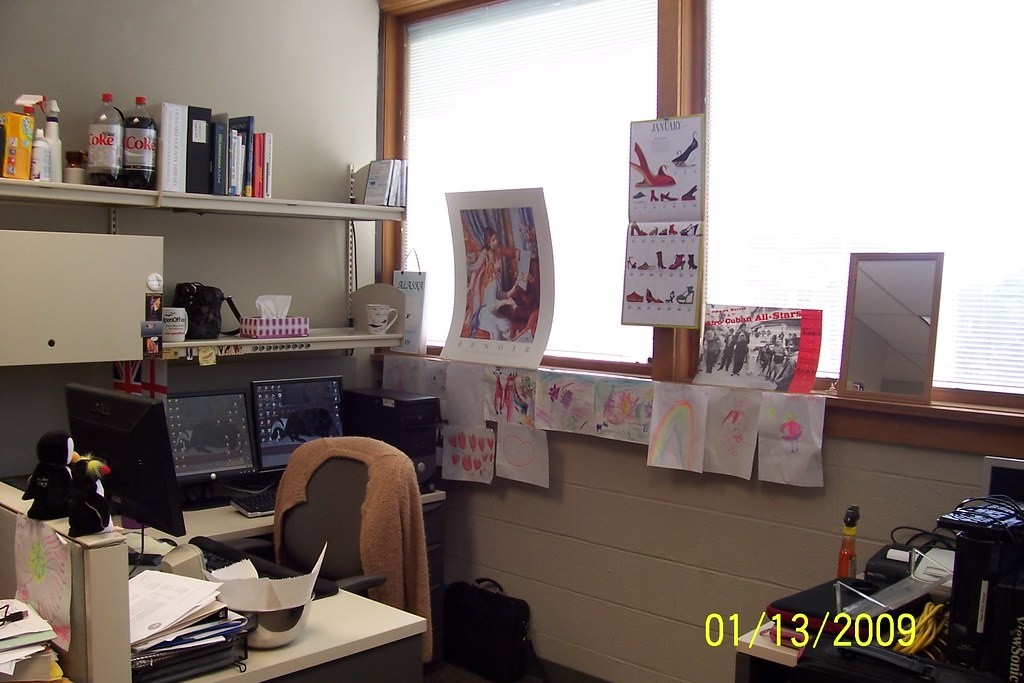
[696,323,800,392]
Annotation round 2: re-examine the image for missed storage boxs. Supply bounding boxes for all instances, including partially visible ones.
[0,112,33,180]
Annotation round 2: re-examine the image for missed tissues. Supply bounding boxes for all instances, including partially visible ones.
[239,294,310,340]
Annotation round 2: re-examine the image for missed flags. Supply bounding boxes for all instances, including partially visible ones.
[112,358,168,401]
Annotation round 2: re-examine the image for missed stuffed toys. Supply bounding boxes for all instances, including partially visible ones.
[21,431,114,537]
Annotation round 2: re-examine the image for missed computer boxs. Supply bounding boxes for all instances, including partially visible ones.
[345,385,440,494]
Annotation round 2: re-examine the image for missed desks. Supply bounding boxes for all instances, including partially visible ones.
[734,572,866,682]
[110,473,458,682]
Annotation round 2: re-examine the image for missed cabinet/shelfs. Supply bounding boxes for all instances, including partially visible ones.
[0,179,413,367]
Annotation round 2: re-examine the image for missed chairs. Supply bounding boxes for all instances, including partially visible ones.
[273,435,432,661]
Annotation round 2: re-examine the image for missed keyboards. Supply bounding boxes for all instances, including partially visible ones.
[936,500,1024,542]
[230,492,278,517]
[196,550,237,574]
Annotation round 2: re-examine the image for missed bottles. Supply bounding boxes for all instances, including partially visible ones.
[88,94,124,188]
[30,128,49,181]
[124,97,157,190]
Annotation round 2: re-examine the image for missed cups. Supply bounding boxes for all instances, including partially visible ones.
[367,304,398,335]
[63,168,85,184]
[162,307,188,342]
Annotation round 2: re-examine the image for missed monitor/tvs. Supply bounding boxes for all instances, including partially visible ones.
[252,375,349,473]
[981,456,1024,503]
[164,388,262,510]
[65,385,186,567]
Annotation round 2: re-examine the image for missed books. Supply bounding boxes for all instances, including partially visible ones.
[363,159,407,206]
[160,101,272,199]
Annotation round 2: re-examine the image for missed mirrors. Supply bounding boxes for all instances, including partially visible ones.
[838,251,943,405]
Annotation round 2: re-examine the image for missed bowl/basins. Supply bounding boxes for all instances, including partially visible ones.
[227,592,316,648]
